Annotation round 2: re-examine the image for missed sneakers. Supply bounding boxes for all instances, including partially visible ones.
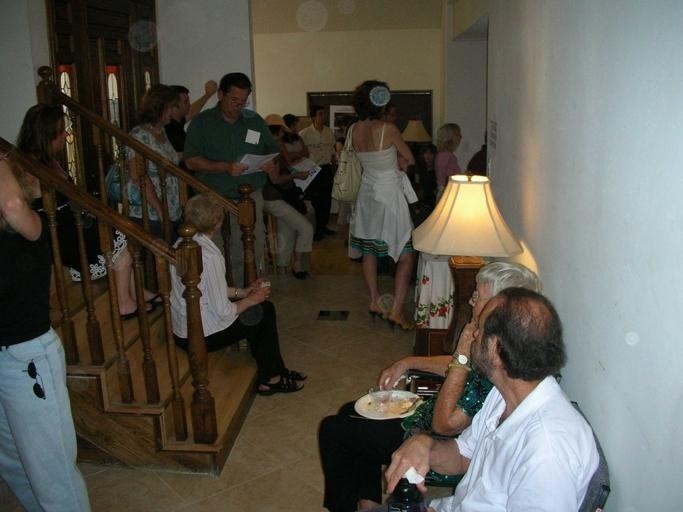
[291,267,309,279]
[318,227,336,236]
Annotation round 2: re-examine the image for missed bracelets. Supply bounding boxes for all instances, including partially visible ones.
[447,363,472,373]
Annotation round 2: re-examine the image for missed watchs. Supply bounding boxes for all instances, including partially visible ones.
[452,352,472,367]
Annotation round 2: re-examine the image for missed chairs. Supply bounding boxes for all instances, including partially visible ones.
[381,401,610,512]
[399,370,562,392]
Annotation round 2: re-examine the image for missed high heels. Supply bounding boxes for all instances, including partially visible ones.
[369,301,389,323]
[387,313,415,332]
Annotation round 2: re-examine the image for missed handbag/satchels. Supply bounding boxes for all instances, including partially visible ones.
[331,148,363,203]
[105,159,141,205]
[278,187,307,215]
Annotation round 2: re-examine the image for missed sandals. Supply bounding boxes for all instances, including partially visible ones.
[258,376,304,396]
[276,368,307,381]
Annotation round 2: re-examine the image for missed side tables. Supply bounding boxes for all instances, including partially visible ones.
[410,328,455,387]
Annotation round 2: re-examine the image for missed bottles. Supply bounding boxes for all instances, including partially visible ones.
[389,478,424,512]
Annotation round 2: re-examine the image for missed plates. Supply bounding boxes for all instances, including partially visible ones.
[354,390,423,421]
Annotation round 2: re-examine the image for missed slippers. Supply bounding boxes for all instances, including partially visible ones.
[119,301,156,321]
[144,293,164,305]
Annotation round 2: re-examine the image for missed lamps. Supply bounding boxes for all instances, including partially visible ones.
[400,119,432,173]
[412,174,524,354]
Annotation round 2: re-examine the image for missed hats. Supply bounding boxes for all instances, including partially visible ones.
[265,114,288,128]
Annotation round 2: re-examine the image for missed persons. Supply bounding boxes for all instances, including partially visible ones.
[168,193,308,396]
[0,156,93,512]
[10,102,164,322]
[384,286,600,512]
[123,72,487,332]
[317,259,543,511]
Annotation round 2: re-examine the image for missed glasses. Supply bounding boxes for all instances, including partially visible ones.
[22,359,46,400]
[471,291,492,302]
[225,95,250,108]
